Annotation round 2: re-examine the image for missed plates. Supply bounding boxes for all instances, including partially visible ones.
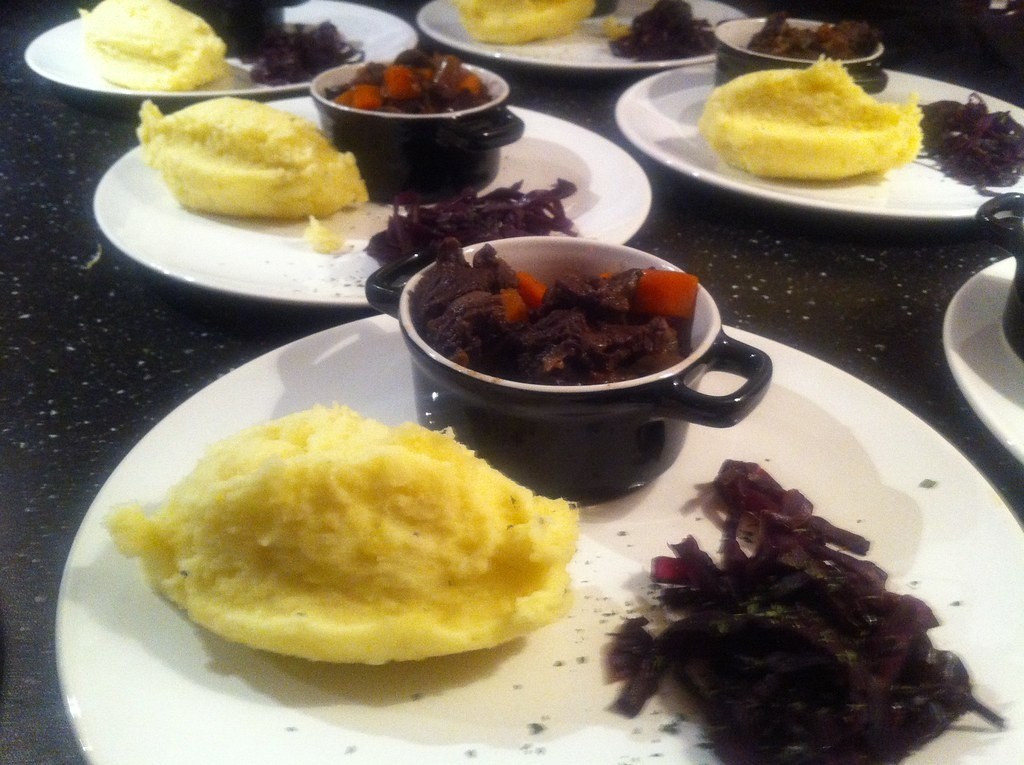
[943,255,1024,465]
[93,95,653,307]
[615,62,1024,221]
[417,0,750,71]
[55,313,1024,765]
[24,0,417,104]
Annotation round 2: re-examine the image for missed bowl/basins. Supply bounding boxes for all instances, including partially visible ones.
[363,236,773,508]
[975,193,1024,360]
[310,61,525,205]
[171,0,309,58]
[712,18,888,94]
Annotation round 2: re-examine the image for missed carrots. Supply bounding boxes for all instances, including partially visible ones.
[335,68,483,111]
[500,264,699,323]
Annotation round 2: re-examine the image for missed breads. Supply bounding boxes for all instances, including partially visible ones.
[77,0,228,91]
[700,55,927,180]
[133,97,365,249]
[452,0,595,46]
[95,404,584,665]
